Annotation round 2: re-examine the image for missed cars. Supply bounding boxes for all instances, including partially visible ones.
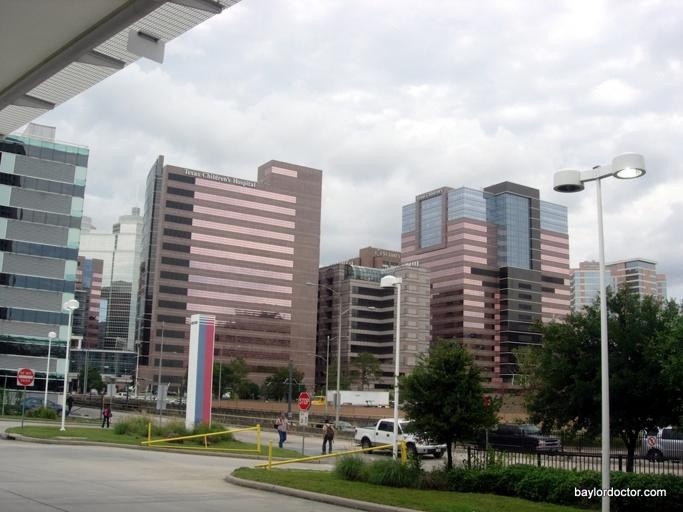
[8,398,69,417]
[477,422,562,454]
[641,424,683,463]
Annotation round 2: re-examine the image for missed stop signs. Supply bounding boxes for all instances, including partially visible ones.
[16,368,35,387]
[297,392,311,410]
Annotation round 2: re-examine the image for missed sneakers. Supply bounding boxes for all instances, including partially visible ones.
[102,425,109,428]
[321,451,332,454]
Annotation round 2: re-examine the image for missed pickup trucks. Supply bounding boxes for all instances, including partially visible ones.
[353,417,447,458]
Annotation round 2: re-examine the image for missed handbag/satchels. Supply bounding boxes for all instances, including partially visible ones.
[273,423,279,428]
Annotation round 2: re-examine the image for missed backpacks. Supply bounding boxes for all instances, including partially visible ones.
[326,424,334,440]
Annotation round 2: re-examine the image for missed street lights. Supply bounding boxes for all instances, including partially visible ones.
[380,276,403,458]
[44,331,57,410]
[554,153,648,511]
[61,299,80,430]
[306,281,376,425]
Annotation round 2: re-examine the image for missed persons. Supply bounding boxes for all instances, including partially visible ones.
[320,419,333,454]
[275,412,288,448]
[100,405,111,427]
[65,394,74,410]
